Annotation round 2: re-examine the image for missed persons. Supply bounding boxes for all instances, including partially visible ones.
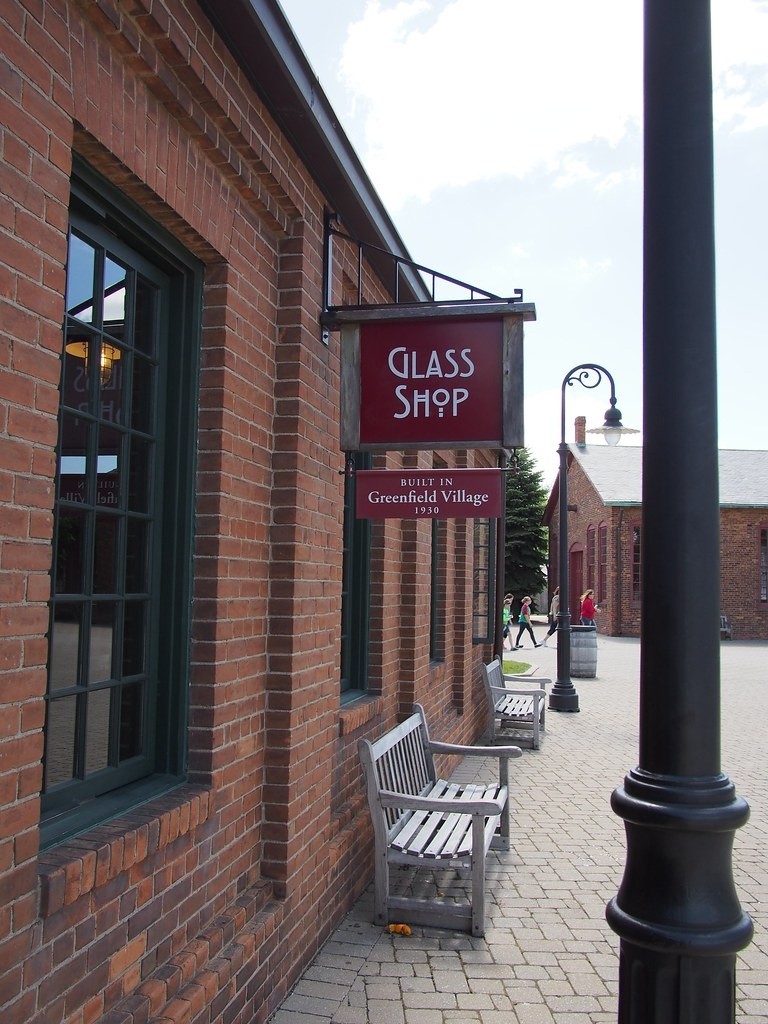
[515,596,543,648]
[503,594,519,651]
[580,589,601,626]
[540,587,560,647]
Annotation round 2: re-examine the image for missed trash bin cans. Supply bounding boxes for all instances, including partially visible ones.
[569,623,599,679]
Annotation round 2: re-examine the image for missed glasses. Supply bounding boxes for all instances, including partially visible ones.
[506,603,511,604]
[526,600,531,601]
[590,594,595,595]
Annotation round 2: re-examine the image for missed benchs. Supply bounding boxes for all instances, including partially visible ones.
[481,654,552,750]
[719,616,733,641]
[357,703,522,936]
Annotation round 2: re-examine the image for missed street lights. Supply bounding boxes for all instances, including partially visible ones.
[548,364,641,713]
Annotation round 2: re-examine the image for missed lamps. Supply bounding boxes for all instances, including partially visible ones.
[66,336,121,385]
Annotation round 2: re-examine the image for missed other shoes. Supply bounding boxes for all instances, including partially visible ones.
[534,644,543,648]
[515,645,523,648]
[539,639,548,647]
[510,647,518,651]
